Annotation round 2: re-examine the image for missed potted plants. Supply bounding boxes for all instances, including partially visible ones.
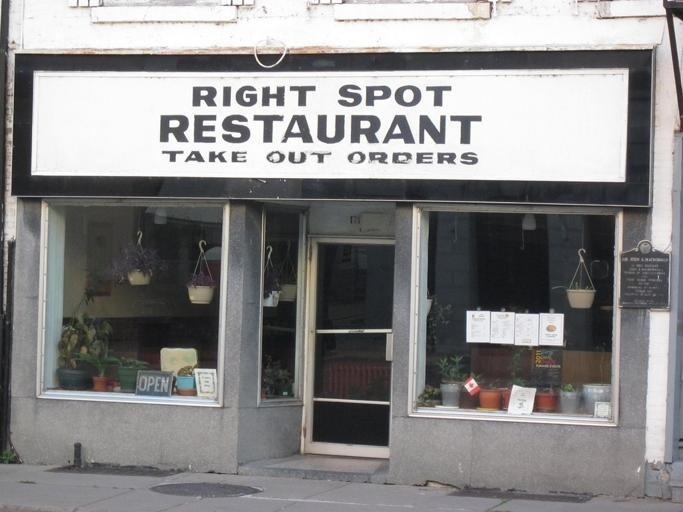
[54,243,297,401]
[417,280,612,416]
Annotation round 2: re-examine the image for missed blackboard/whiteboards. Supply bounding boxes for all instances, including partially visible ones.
[618,240,672,312]
[136,369,175,396]
